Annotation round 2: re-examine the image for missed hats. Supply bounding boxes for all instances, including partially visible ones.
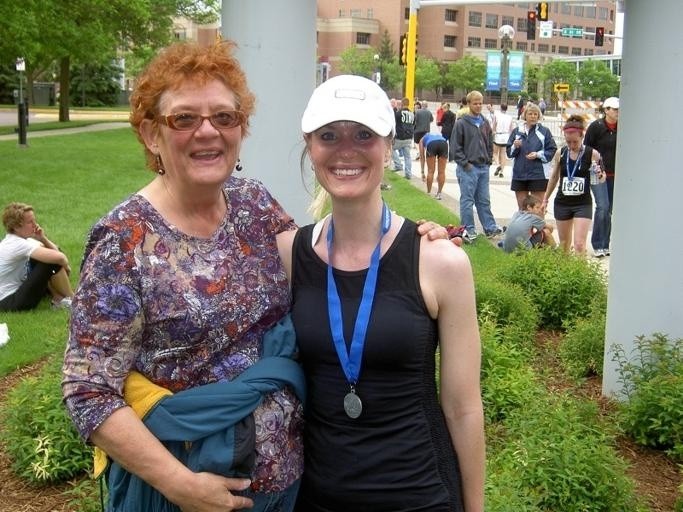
[298,72,401,141]
[600,96,621,110]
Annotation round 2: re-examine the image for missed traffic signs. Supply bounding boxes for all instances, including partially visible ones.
[555,85,570,92]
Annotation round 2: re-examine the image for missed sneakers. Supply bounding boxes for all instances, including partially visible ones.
[427,192,431,196]
[499,173,504,177]
[51,296,73,310]
[434,192,442,200]
[604,248,609,256]
[494,165,501,175]
[594,249,606,258]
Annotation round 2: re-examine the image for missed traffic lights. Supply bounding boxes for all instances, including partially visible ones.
[539,2,548,21]
[400,35,408,63]
[594,27,605,46]
[527,12,536,41]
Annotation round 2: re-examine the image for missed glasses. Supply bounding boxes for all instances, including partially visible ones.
[148,106,247,133]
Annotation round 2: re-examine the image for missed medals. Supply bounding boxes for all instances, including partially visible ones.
[324,198,391,420]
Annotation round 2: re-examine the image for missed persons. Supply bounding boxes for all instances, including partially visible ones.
[0,201,74,312]
[390,90,619,256]
[63,34,464,512]
[271,74,487,512]
